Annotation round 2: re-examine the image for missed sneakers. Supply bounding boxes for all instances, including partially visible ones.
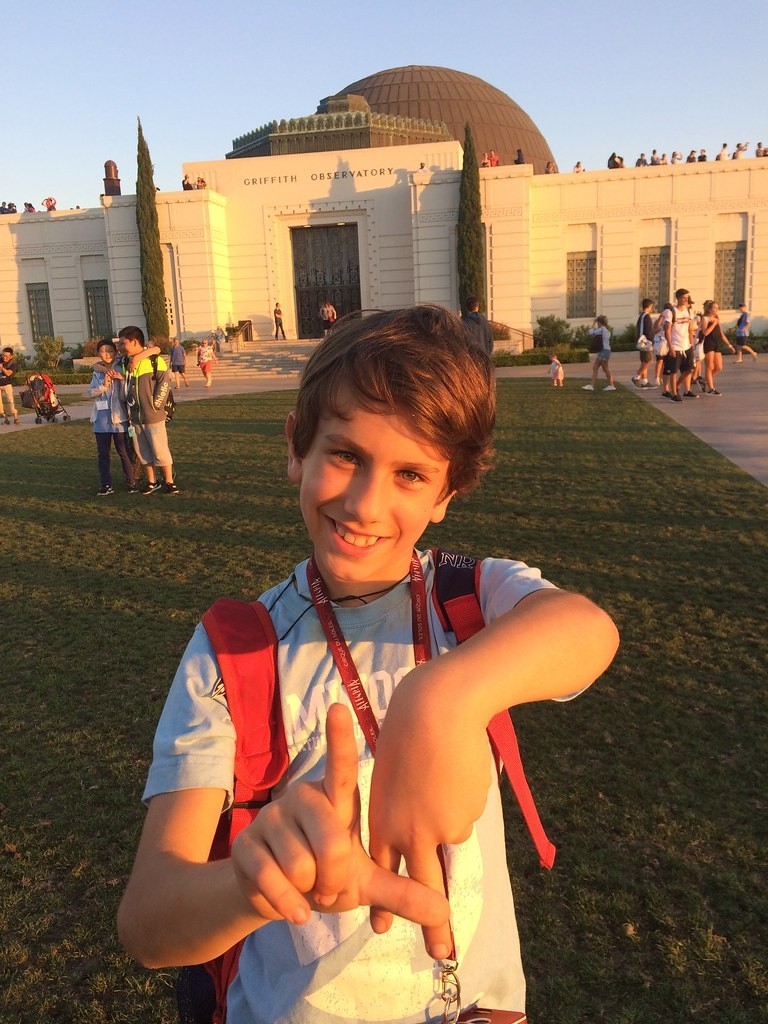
[629,376,641,389]
[96,484,114,496]
[166,482,179,494]
[691,377,696,384]
[128,485,139,493]
[662,391,672,398]
[696,378,706,393]
[698,376,703,381]
[581,384,595,391]
[603,385,616,390]
[682,391,700,399]
[706,388,722,396]
[670,396,683,404]
[142,480,161,494]
[641,384,658,389]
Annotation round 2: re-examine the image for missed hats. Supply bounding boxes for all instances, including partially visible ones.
[688,295,695,304]
[174,337,178,340]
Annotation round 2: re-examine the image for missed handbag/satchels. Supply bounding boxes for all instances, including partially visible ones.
[589,326,603,353]
[636,334,654,352]
[20,387,34,408]
[694,343,705,361]
[652,307,676,356]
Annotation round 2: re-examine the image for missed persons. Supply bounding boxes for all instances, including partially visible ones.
[211,326,226,353]
[631,289,736,404]
[548,353,565,387]
[274,302,286,341]
[320,301,336,335]
[0,347,21,425]
[168,337,189,389]
[417,163,429,173]
[197,338,218,388]
[0,174,207,215]
[461,296,494,359]
[86,326,179,496]
[581,314,616,391]
[117,300,620,1024]
[482,141,768,174]
[728,303,758,364]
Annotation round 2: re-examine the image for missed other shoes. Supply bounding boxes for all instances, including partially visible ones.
[174,386,181,388]
[4,420,9,425]
[13,419,20,424]
[186,383,189,387]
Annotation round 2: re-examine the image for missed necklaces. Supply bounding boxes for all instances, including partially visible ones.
[209,568,410,699]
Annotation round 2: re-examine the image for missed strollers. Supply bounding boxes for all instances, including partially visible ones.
[24,371,70,424]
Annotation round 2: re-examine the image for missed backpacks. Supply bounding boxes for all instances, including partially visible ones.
[124,354,176,424]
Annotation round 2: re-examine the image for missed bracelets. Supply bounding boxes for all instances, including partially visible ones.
[728,345,732,349]
